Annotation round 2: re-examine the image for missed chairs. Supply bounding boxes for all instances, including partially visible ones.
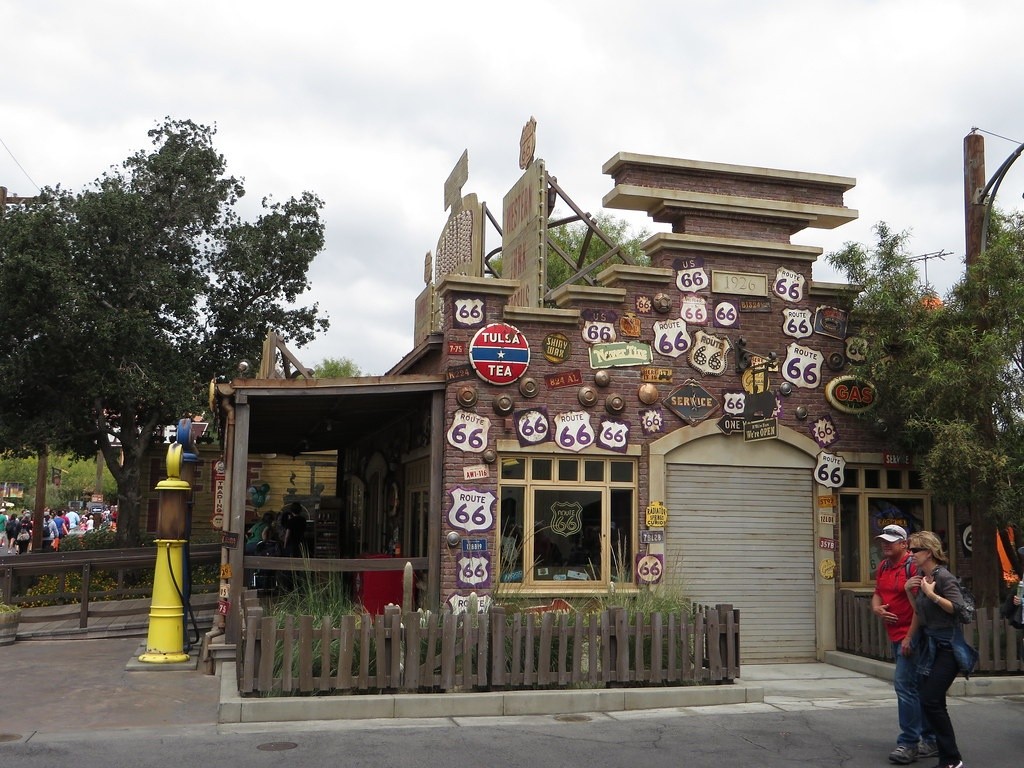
[251,539,282,592]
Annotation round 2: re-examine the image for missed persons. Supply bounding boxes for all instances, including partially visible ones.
[245,502,306,596]
[0,506,118,555]
[904,531,979,768]
[871,525,939,764]
[1002,547,1024,628]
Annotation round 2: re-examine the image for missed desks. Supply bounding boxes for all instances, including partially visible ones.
[353,554,416,625]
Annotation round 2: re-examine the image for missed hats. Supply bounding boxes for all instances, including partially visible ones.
[873,524,907,543]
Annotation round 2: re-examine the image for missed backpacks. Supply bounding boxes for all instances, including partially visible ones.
[42,521,54,537]
[17,527,30,541]
[930,565,977,624]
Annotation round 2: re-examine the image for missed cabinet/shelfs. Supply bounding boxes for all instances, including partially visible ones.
[314,509,340,594]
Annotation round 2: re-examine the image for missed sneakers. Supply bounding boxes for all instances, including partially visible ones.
[889,743,918,763]
[8,551,12,554]
[915,741,940,758]
[939,760,963,768]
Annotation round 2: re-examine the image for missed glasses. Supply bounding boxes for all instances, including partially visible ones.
[878,529,905,539]
[907,547,929,554]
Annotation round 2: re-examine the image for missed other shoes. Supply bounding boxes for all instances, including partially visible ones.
[0,546,3,549]
[2,542,5,545]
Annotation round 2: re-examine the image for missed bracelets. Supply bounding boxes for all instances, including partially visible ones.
[934,595,941,604]
